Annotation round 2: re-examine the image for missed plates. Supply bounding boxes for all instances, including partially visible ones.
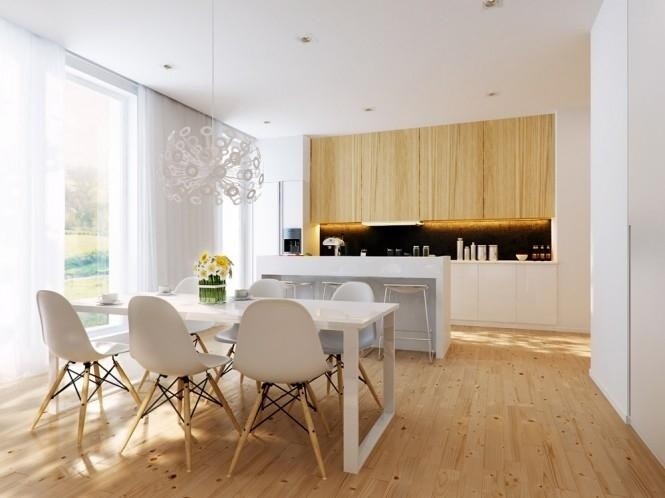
[95,301,126,305]
[401,253,410,256]
[154,292,176,296]
[228,297,256,301]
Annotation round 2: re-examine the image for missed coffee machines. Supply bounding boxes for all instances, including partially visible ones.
[278,227,302,255]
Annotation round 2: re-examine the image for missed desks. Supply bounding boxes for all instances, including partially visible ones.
[49,293,399,474]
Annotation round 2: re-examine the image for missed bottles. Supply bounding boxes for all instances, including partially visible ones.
[396,249,402,256]
[471,242,476,262]
[540,244,546,260]
[546,244,551,260]
[532,244,536,260]
[361,250,368,256]
[465,245,469,262]
[386,249,394,256]
[456,238,464,261]
[536,244,539,260]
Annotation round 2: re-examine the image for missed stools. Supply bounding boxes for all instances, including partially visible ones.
[379,284,433,361]
[281,280,315,299]
[322,281,375,358]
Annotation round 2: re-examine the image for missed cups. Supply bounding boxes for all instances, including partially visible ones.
[277,281,294,297]
[477,245,485,260]
[235,289,249,297]
[293,283,315,313]
[338,240,347,256]
[159,286,172,292]
[99,294,118,303]
[423,246,429,256]
[488,245,497,261]
[321,281,344,319]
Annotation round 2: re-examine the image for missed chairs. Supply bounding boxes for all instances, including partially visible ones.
[226,299,333,480]
[119,296,249,472]
[204,279,294,405]
[138,277,220,392]
[31,290,142,449]
[318,281,382,410]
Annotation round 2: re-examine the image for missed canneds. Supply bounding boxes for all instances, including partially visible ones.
[477,245,487,260]
[395,248,403,256]
[423,246,429,256]
[387,249,395,256]
[488,244,498,261]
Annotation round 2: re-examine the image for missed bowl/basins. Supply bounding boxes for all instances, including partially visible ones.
[516,254,528,261]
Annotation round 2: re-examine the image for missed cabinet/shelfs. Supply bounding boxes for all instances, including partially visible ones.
[311,127,419,223]
[418,114,556,220]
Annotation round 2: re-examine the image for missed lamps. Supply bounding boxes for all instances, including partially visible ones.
[159,1,264,207]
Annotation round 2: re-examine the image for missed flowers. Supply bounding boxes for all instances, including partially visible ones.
[193,252,234,279]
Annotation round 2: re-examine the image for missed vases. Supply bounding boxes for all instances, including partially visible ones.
[200,280,226,304]
